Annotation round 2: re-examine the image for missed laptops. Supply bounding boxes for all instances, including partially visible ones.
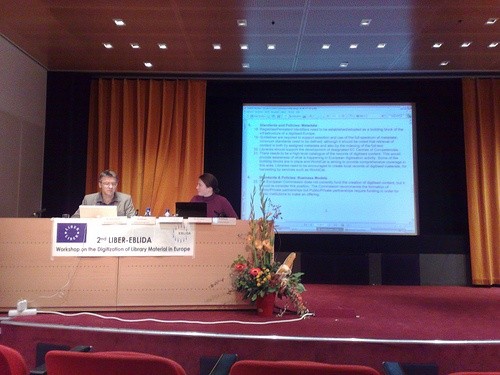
[79,205,118,219]
[176,201,207,219]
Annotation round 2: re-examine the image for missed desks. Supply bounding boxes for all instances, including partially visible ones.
[0,217,116,311]
[116,219,274,311]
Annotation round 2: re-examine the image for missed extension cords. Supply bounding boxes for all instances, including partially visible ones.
[8,308,38,315]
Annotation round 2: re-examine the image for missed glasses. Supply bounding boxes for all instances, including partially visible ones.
[101,182,117,187]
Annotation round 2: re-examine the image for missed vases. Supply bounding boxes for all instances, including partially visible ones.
[256,292,277,317]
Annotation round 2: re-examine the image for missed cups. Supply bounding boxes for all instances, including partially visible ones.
[62,214,70,218]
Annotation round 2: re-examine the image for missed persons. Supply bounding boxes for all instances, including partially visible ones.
[71,170,136,218]
[190,173,239,219]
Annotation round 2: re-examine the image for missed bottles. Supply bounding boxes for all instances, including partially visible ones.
[163,208,170,217]
[144,207,151,217]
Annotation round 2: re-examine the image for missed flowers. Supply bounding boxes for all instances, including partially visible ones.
[228,174,311,319]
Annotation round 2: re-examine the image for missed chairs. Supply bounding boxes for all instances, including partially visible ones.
[0,344,500,375]
